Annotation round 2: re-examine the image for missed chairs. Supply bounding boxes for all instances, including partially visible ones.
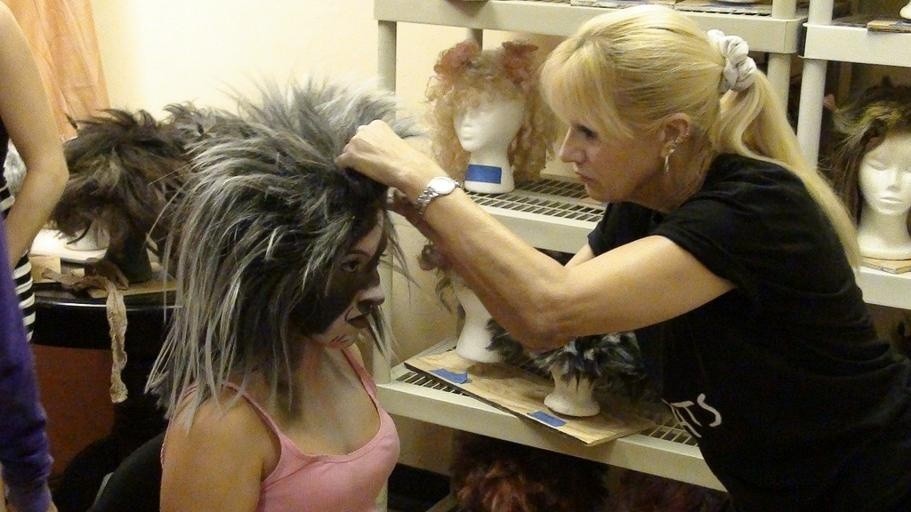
[95,431,167,512]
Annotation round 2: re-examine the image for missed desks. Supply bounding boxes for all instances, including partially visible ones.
[13,262,176,479]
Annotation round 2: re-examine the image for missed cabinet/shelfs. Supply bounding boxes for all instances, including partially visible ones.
[371,0,803,512]
[795,0,910,318]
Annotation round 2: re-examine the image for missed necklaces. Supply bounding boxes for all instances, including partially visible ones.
[642,147,715,211]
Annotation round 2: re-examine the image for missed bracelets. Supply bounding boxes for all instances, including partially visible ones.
[410,214,421,225]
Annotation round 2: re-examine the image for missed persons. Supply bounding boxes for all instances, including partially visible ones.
[418,241,509,363]
[334,4,911,512]
[449,432,731,512]
[819,80,911,264]
[140,75,443,511]
[424,37,561,194]
[1,216,58,511]
[51,105,180,283]
[484,314,644,417]
[1,2,71,344]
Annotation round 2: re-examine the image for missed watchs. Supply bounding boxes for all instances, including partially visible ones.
[413,176,462,218]
